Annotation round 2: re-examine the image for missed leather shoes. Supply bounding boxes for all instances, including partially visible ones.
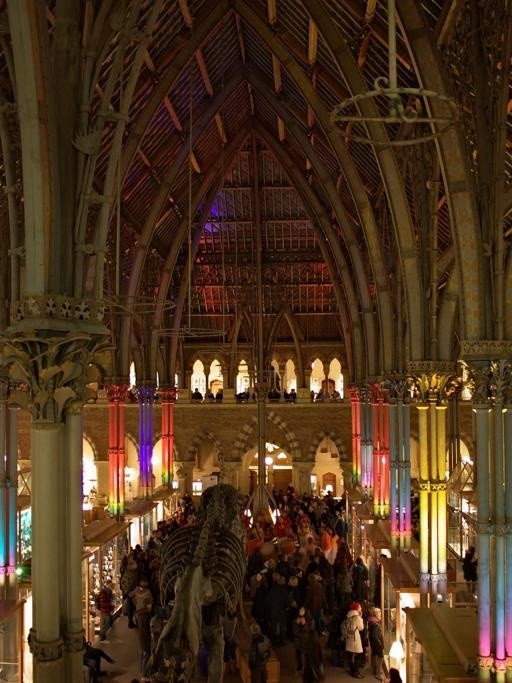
[353,673,363,677]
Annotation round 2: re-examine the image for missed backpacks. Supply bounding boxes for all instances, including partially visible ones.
[341,614,358,640]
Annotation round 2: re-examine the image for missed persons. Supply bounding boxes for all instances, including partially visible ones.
[462,547,477,594]
[98,485,403,682]
[192,388,341,402]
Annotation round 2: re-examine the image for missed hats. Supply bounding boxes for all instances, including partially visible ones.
[351,601,359,609]
